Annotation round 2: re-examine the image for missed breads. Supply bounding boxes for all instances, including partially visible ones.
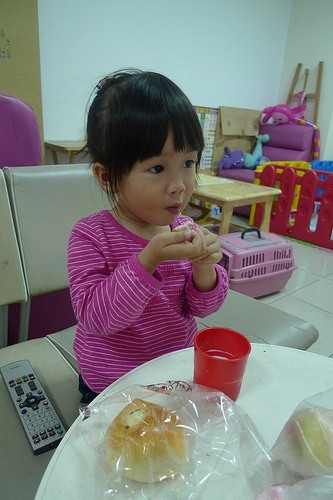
[104,398,187,483]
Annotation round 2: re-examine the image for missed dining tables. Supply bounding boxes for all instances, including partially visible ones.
[34,343,333,500]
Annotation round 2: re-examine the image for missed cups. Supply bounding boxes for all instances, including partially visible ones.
[193,327,251,403]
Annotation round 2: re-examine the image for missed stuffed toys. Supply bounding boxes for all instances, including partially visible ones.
[218,132,270,172]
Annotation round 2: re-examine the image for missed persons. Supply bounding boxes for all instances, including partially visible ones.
[67,68,228,405]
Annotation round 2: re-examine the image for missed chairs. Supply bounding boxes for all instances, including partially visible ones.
[0,163,319,500]
[218,124,315,216]
[1,94,78,346]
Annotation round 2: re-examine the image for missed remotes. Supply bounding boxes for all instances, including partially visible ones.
[0,359,67,456]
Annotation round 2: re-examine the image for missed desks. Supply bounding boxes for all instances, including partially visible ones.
[192,173,282,235]
[45,141,87,165]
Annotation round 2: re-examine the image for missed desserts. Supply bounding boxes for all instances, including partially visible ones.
[174,221,196,243]
